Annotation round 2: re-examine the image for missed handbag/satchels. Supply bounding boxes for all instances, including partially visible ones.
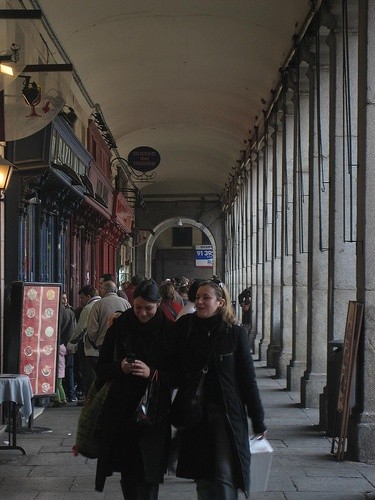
[167,368,209,415]
[75,375,112,460]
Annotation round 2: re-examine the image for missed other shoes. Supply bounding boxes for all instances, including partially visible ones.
[53,401,61,407]
[62,400,68,406]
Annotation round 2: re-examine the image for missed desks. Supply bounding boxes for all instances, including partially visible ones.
[0,373,33,455]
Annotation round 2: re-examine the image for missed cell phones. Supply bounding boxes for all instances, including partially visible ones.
[126,353,135,363]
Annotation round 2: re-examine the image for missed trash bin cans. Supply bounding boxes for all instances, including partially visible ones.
[322,342,348,437]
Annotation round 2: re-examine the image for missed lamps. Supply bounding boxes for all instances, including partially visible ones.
[19,75,42,107]
[0,42,21,76]
[0,154,19,202]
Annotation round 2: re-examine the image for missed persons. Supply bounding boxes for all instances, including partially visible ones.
[176,279,269,499]
[95,279,186,500]
[55,273,252,408]
